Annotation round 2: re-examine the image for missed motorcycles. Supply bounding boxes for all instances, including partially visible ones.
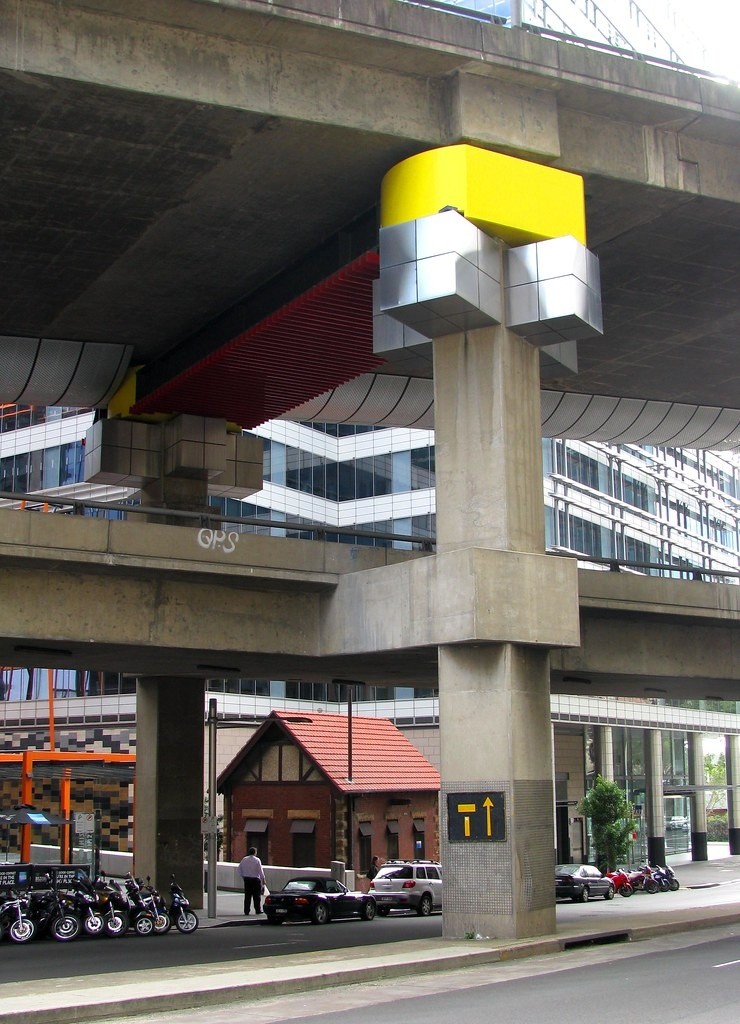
[32,869,81,942]
[60,866,105,937]
[139,876,172,934]
[625,860,659,894]
[91,870,131,938]
[603,868,633,897]
[0,875,40,944]
[119,871,155,937]
[650,864,670,892]
[663,864,679,891]
[164,876,199,933]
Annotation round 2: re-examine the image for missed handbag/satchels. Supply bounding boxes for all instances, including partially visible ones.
[263,884,270,897]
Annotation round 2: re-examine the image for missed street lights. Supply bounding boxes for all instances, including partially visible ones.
[208,698,314,918]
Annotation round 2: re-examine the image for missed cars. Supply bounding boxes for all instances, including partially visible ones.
[554,864,616,903]
[263,877,377,924]
[682,817,688,833]
[666,815,684,831]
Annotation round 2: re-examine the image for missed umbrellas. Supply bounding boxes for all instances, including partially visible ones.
[0,805,72,862]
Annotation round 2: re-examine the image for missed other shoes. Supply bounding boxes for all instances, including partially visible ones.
[256,911,263,915]
[245,911,249,916]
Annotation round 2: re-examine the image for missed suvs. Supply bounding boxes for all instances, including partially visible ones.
[368,859,442,917]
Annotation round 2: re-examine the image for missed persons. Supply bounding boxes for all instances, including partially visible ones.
[237,847,266,915]
[367,856,378,880]
